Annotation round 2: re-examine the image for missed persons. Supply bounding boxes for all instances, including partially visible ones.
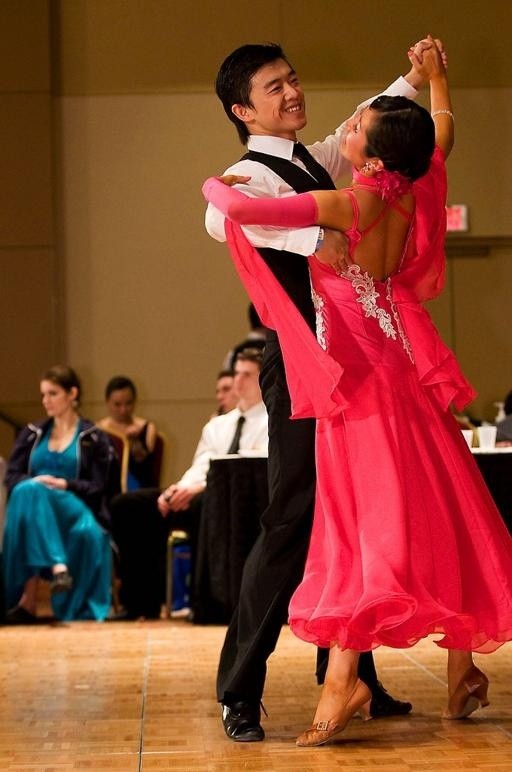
[494,388,512,448]
[93,375,157,492]
[222,301,277,370]
[215,371,236,414]
[114,348,270,625]
[1,366,121,623]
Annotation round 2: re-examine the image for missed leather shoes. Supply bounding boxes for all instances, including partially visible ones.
[369,680,411,716]
[105,609,142,621]
[222,700,268,742]
[50,572,71,592]
[1,605,38,624]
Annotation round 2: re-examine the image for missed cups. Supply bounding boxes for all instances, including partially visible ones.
[459,428,473,450]
[477,426,498,452]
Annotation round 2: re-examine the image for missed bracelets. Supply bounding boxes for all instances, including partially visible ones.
[432,107,456,122]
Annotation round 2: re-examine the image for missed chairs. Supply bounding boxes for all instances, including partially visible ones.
[0,414,22,462]
[97,425,130,613]
[146,432,164,488]
[165,528,190,618]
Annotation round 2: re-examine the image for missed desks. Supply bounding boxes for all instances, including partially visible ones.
[192,453,511,625]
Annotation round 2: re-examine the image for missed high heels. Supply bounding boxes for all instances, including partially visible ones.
[296,676,373,747]
[442,664,490,720]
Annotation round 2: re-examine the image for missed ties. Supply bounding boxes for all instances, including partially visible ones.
[293,141,337,190]
[228,417,245,454]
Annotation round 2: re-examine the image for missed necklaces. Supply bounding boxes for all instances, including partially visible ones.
[351,164,377,186]
